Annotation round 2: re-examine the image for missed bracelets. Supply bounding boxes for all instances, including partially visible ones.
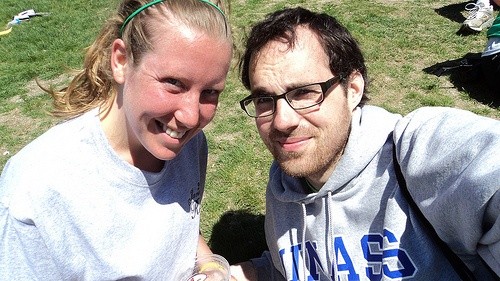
[198,261,226,273]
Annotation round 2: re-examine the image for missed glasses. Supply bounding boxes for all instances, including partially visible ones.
[240,70,348,119]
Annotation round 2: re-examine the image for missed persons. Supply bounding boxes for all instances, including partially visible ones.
[0,0,238,281]
[228,6,500,281]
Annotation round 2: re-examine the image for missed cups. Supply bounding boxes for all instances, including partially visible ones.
[174,254,232,281]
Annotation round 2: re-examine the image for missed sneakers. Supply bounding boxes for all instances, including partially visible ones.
[462,3,495,32]
